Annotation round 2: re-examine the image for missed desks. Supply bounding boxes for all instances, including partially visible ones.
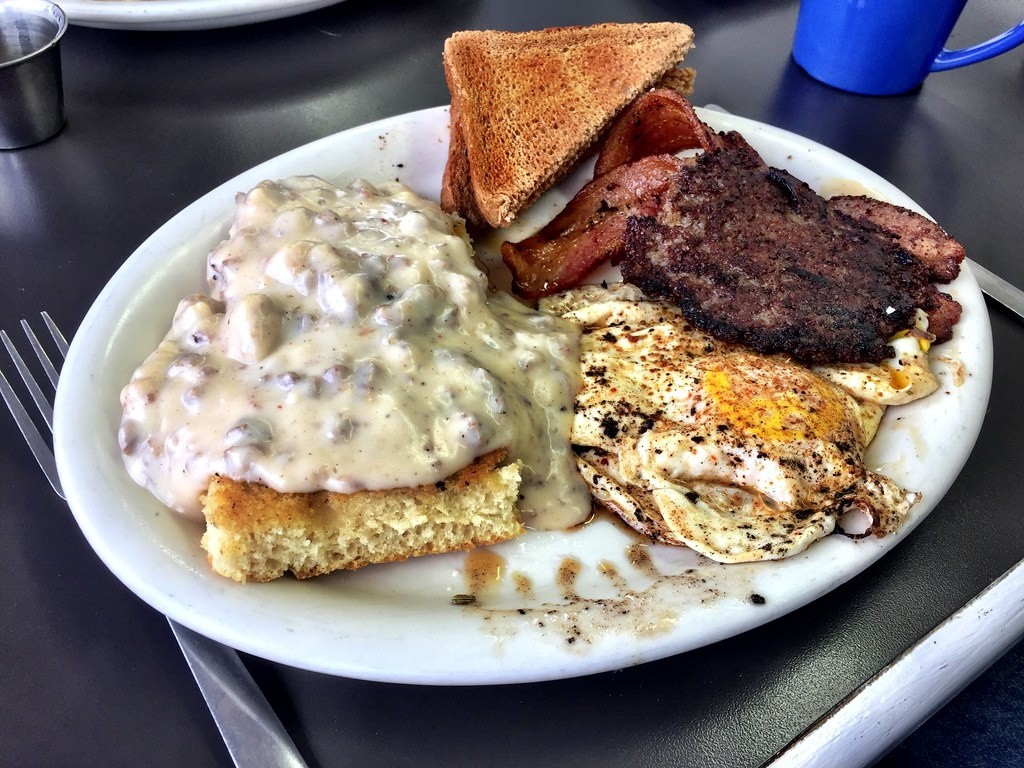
[0,0,1024,768]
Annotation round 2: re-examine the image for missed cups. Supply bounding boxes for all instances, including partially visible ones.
[1,1,69,150]
[793,1,1024,96]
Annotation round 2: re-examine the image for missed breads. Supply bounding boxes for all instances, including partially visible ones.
[198,449,531,583]
[440,21,698,241]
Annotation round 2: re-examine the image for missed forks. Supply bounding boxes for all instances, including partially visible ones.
[0,312,310,768]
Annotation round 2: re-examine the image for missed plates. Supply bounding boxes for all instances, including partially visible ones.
[54,0,344,32]
[53,106,994,686]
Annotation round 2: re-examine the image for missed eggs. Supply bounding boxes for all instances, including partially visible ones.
[537,283,941,563]
